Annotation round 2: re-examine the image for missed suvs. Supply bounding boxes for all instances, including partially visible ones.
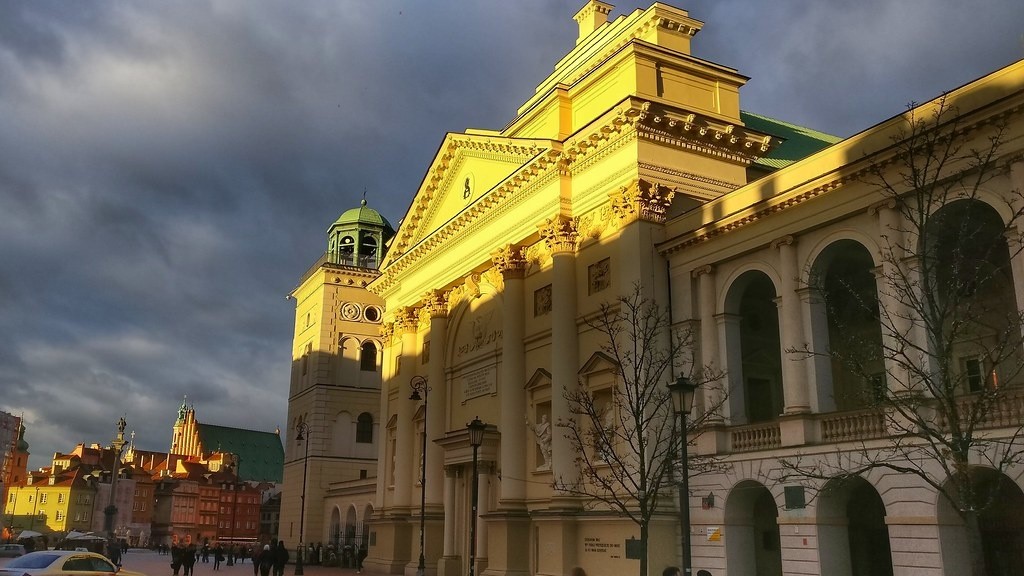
[0,544,25,558]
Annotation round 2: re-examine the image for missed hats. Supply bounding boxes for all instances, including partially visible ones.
[264,544,269,550]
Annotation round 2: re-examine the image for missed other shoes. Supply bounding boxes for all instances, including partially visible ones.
[357,571,360,573]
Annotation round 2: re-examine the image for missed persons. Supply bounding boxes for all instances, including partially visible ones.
[251,547,262,576]
[293,541,367,576]
[17,535,129,565]
[571,567,586,576]
[662,566,680,576]
[259,545,273,576]
[696,569,712,576]
[272,540,289,576]
[157,543,247,576]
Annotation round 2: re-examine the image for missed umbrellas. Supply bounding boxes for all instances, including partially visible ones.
[16,530,44,540]
[66,530,85,538]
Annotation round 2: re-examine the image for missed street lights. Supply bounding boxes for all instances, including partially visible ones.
[666,373,699,576]
[226,453,241,566]
[410,375,426,576]
[295,421,310,575]
[465,416,487,574]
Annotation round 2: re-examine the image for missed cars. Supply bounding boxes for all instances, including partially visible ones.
[0,550,147,576]
